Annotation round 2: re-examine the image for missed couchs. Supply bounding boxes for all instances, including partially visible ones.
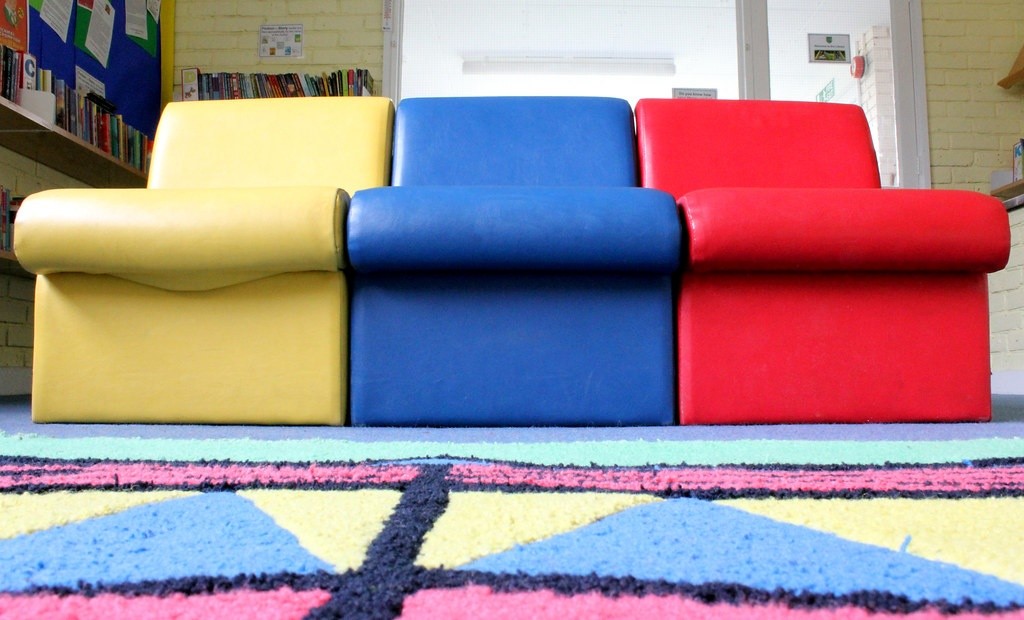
[632,98,1011,429]
[13,97,399,427]
[347,94,688,429]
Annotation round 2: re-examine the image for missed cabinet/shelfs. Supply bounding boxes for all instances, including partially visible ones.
[0,98,148,272]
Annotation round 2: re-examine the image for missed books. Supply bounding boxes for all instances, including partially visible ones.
[0,185,26,252]
[181,67,373,102]
[0,44,154,175]
